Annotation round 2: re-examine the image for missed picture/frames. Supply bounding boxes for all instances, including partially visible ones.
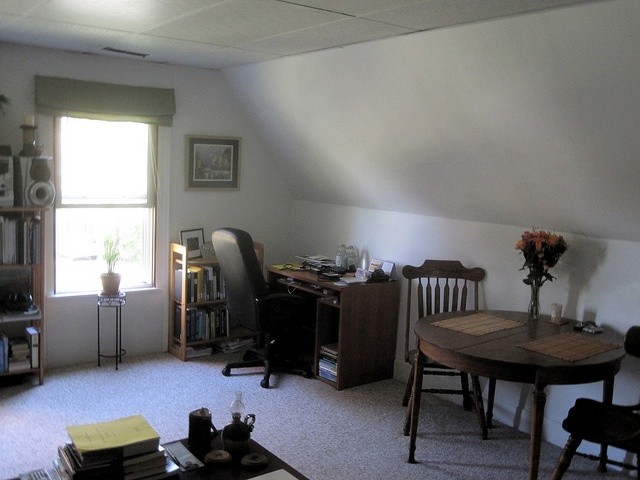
[184,133,242,192]
[177,227,205,261]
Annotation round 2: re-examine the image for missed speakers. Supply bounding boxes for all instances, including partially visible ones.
[19,155,56,206]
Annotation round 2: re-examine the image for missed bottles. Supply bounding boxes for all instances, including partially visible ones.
[344,245,356,268]
[335,243,346,269]
[361,250,368,271]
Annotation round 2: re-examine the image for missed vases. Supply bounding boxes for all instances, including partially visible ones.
[526,280,544,321]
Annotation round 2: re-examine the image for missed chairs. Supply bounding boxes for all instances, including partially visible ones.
[210,226,313,388]
[403,259,490,442]
[549,325,640,480]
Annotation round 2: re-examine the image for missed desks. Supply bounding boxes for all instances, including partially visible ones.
[407,309,627,480]
[8,428,311,480]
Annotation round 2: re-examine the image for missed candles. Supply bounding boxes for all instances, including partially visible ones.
[97,238,124,297]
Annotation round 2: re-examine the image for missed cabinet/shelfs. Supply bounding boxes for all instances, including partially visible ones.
[0,206,46,386]
[264,264,401,390]
[168,242,266,362]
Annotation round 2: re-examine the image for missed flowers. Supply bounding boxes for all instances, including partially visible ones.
[515,228,568,318]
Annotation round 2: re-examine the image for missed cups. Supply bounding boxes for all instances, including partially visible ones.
[550,304,562,323]
[188,407,219,449]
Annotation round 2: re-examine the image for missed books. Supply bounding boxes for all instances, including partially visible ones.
[52,415,180,480]
[173,339,256,359]
[1,326,41,373]
[318,345,338,383]
[0,216,43,266]
[333,277,365,288]
[173,306,230,344]
[174,265,229,304]
[294,254,336,268]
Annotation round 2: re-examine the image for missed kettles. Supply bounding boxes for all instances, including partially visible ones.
[221,391,256,451]
